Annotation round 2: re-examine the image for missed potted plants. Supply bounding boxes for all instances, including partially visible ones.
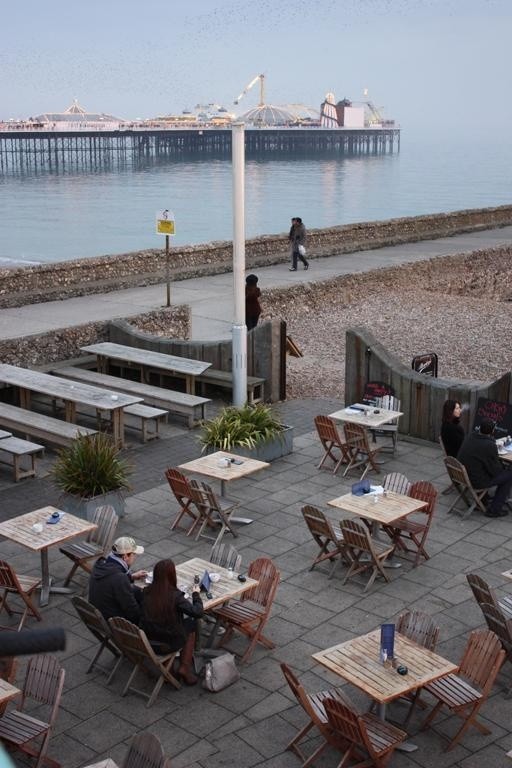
[193,403,294,463]
[50,428,140,522]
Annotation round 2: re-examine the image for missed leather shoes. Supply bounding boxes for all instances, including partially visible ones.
[485,508,508,518]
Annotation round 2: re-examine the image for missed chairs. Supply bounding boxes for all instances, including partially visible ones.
[122,732,170,768]
[280,662,360,768]
[437,434,455,495]
[0,559,42,632]
[372,395,401,457]
[212,558,280,666]
[68,596,125,684]
[422,631,505,753]
[442,456,491,520]
[342,422,384,482]
[313,415,351,474]
[299,504,353,580]
[59,505,119,587]
[204,543,242,647]
[368,611,440,722]
[385,480,440,567]
[0,654,66,768]
[187,479,240,549]
[164,469,218,530]
[338,519,394,593]
[108,617,183,708]
[465,572,512,664]
[323,697,407,768]
[379,472,411,551]
[79,758,122,768]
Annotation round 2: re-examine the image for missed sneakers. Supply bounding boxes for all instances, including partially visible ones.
[304,263,309,270]
[289,267,297,271]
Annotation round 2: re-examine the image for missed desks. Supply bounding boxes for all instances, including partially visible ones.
[310,624,460,722]
[0,505,99,607]
[176,451,271,532]
[133,557,260,657]
[80,341,213,395]
[0,675,23,704]
[327,485,429,562]
[492,438,512,462]
[328,402,405,470]
[0,363,144,449]
[502,565,512,583]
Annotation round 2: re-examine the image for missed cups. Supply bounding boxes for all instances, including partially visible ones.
[364,411,371,418]
[145,571,153,583]
[209,573,220,583]
[33,524,43,533]
[110,390,119,401]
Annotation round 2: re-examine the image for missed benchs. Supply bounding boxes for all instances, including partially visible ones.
[0,428,46,483]
[0,401,98,455]
[53,364,212,428]
[122,404,169,444]
[114,360,267,405]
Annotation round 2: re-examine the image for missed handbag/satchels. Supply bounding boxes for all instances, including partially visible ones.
[201,652,240,692]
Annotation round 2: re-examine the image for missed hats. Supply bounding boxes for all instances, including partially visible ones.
[112,537,145,554]
[246,274,258,284]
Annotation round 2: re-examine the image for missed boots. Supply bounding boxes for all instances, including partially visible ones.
[177,631,199,686]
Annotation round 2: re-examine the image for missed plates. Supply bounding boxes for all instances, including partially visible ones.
[345,408,361,414]
[184,593,189,599]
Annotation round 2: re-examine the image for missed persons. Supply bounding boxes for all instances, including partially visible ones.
[246,274,261,331]
[137,559,203,685]
[88,537,148,627]
[290,217,309,272]
[288,218,297,270]
[458,419,511,518]
[441,399,465,457]
